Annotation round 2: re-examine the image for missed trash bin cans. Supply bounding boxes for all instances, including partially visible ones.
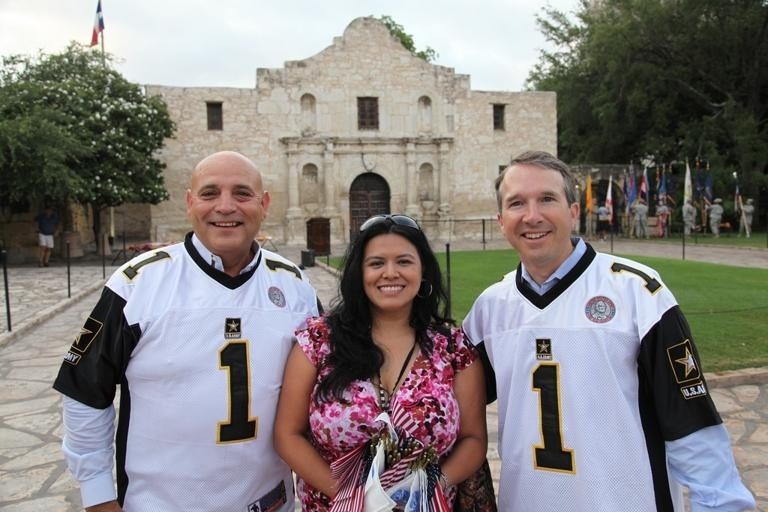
[302,217,330,266]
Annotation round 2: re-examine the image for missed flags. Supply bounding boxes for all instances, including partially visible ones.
[90,0,104,47]
[586,161,741,224]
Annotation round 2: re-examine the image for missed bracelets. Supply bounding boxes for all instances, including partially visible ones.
[441,472,448,494]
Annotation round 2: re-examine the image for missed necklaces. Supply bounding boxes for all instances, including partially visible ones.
[377,333,417,412]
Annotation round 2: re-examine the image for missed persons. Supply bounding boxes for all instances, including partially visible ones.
[584,197,754,239]
[460,150,755,512]
[53,151,324,512]
[34,205,60,267]
[273,213,497,512]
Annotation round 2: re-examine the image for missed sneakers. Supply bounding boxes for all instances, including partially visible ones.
[39,262,49,267]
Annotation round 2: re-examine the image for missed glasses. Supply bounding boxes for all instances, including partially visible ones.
[359,213,421,231]
